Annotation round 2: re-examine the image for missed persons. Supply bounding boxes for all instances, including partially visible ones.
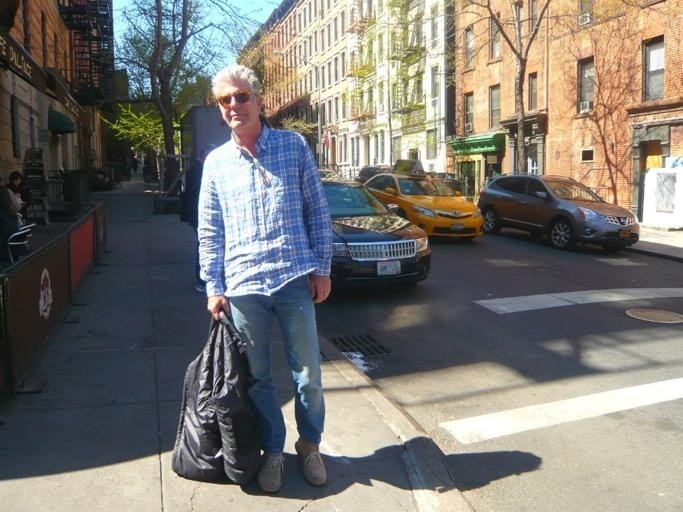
[0,171,31,262]
[196,62,334,493]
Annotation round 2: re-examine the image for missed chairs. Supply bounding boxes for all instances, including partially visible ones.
[7,223,35,265]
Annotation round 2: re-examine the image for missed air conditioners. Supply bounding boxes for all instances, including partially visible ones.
[579,13,591,25]
[580,101,590,114]
[465,123,472,132]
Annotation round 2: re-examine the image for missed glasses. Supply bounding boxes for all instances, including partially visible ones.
[213,90,258,109]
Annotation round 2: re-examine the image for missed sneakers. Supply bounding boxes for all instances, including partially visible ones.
[295,435,327,487]
[256,450,285,492]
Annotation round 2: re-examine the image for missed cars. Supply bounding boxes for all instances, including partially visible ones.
[356,165,392,183]
[365,157,484,244]
[321,178,432,290]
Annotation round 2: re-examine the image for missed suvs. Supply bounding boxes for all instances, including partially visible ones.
[477,171,639,252]
